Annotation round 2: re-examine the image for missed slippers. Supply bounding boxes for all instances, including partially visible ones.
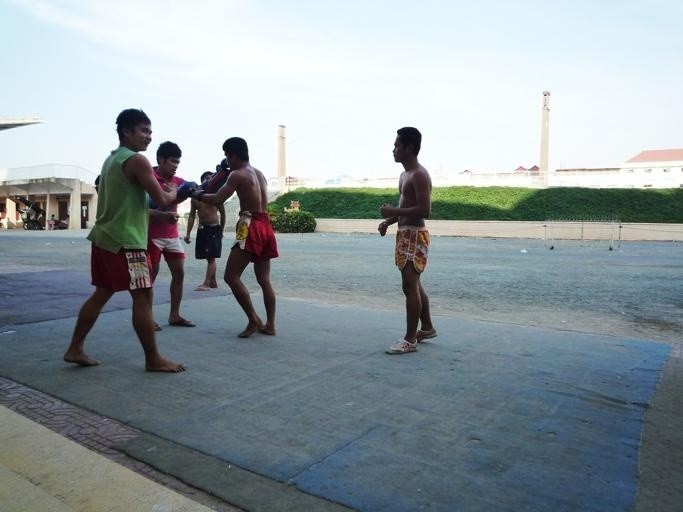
[169,317,196,327]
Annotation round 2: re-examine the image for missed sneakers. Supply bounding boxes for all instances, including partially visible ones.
[416,329,438,342]
[385,338,418,354]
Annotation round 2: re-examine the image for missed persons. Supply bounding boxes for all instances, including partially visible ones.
[30,211,69,229]
[190,137,281,337]
[64,108,188,373]
[378,126,439,355]
[146,141,232,331]
[184,171,226,291]
